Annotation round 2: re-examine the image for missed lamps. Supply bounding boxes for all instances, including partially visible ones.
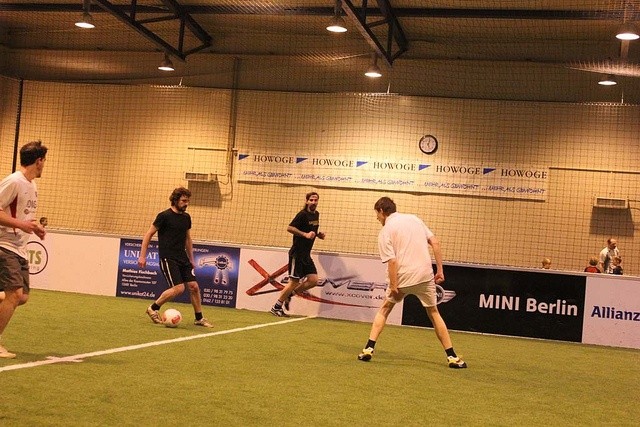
[75,1,96,28]
[325,0,347,32]
[597,73,617,86]
[615,9,639,41]
[364,50,382,78]
[157,51,175,72]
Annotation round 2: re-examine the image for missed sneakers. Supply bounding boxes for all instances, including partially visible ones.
[270,308,289,319]
[146,305,164,327]
[284,304,291,317]
[359,348,373,359]
[0,345,15,359]
[448,356,467,368]
[194,318,214,327]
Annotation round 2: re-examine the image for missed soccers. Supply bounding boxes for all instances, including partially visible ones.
[163,309,182,329]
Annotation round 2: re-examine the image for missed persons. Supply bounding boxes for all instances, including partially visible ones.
[358,196,467,368]
[599,238,621,273]
[0,139,48,359]
[541,258,554,270]
[138,187,214,328]
[613,256,623,275]
[583,257,601,274]
[39,217,48,226]
[269,192,326,318]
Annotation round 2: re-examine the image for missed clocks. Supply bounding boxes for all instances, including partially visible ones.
[419,134,438,154]
